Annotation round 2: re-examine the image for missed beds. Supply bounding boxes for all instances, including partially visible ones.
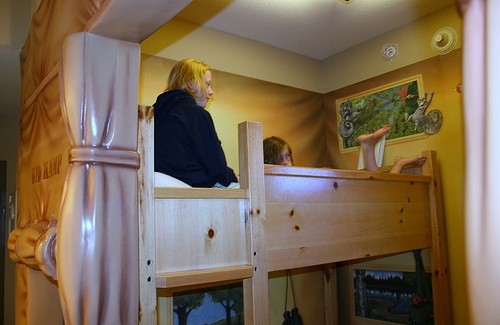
[139,106,454,325]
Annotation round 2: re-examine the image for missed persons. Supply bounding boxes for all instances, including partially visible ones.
[154,57,240,187]
[263,122,426,174]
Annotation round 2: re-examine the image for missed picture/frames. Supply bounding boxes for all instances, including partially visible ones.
[335,74,443,155]
[167,279,246,325]
[348,264,435,325]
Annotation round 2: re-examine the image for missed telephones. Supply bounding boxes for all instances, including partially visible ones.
[9,192,15,221]
